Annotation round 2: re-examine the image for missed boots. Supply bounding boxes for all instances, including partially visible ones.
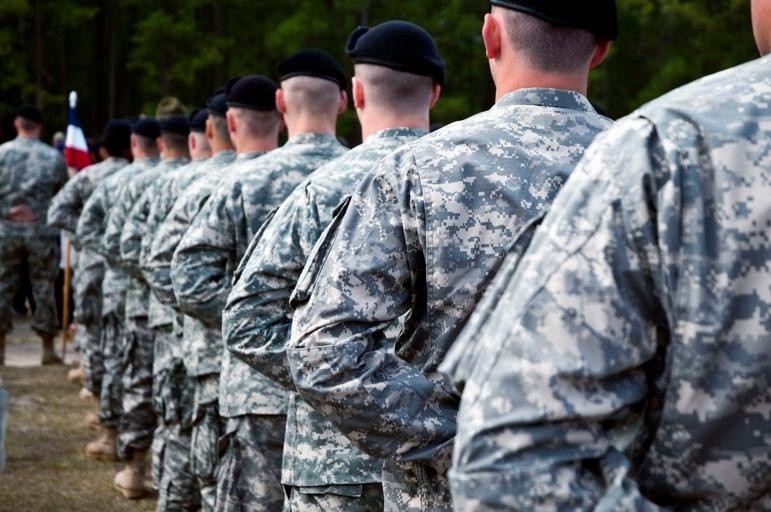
[113,448,145,499]
[41,335,62,364]
[65,366,118,461]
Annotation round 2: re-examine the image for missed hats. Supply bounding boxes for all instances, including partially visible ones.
[225,74,277,113]
[19,105,45,125]
[276,50,348,90]
[491,1,619,42]
[346,20,445,84]
[93,94,230,146]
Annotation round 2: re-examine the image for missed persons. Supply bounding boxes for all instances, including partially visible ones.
[45,48,358,512]
[285,0,626,512]
[1,104,70,365]
[445,0,771,511]
[220,17,442,512]
